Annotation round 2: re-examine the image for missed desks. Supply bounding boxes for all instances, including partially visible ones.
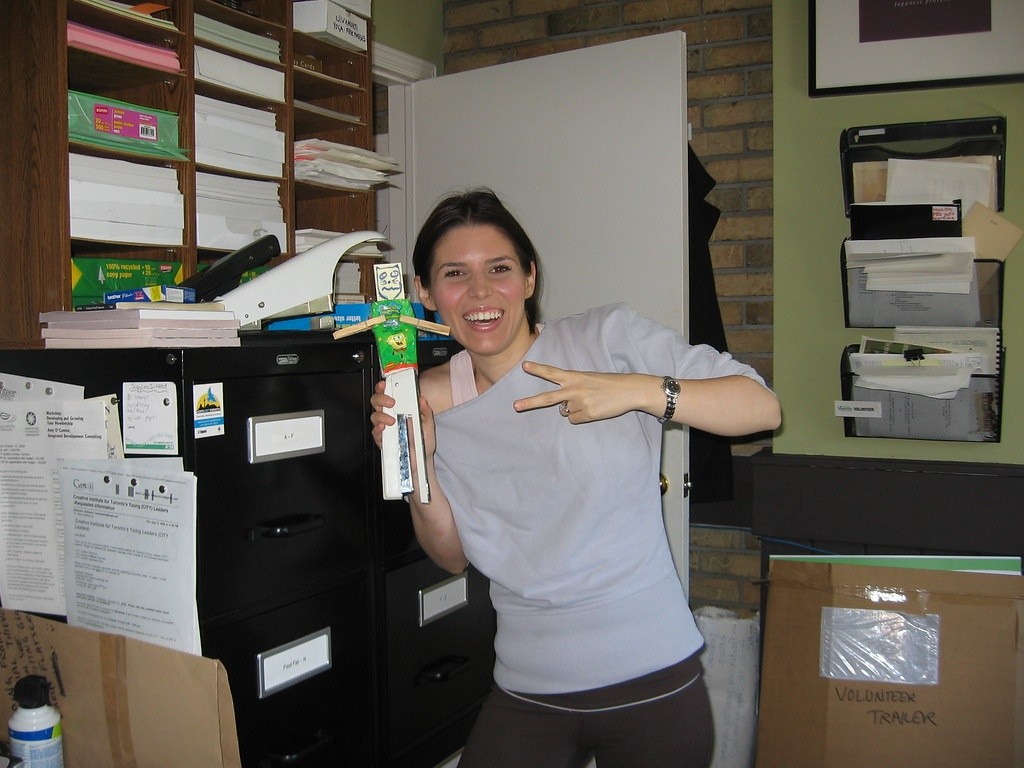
[0,313,498,768]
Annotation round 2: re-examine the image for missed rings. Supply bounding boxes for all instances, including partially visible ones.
[559,402,570,412]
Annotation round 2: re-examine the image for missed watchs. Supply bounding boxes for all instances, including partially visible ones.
[657,376,681,424]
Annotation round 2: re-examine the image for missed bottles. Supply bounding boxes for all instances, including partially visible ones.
[7,674,65,768]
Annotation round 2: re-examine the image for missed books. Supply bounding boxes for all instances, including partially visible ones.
[39,302,241,349]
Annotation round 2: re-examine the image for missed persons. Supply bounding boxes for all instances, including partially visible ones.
[370,186,782,768]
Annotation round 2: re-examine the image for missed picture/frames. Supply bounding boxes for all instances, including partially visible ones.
[806,0,1024,97]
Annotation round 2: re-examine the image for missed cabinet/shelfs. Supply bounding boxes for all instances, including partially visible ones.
[0,0,376,350]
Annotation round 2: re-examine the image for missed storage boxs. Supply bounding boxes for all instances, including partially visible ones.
[67,90,183,156]
[70,261,184,312]
[194,45,289,102]
[756,563,1024,768]
[293,0,371,51]
